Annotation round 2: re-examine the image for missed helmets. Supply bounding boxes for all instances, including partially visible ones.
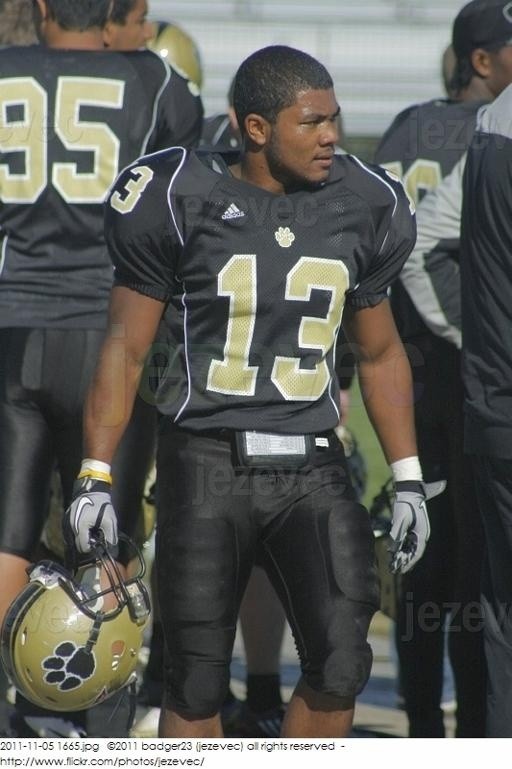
[0,534,152,714]
[144,20,202,96]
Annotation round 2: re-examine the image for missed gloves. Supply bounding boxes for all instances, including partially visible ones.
[62,477,118,555]
[389,481,430,576]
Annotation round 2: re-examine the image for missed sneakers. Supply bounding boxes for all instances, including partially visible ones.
[244,702,290,738]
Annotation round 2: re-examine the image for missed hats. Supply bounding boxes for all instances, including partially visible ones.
[452,2,512,52]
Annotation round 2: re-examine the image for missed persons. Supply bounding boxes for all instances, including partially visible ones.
[375,0,512,738]
[459,81,512,738]
[102,0,154,52]
[1,0,204,738]
[61,45,430,738]
